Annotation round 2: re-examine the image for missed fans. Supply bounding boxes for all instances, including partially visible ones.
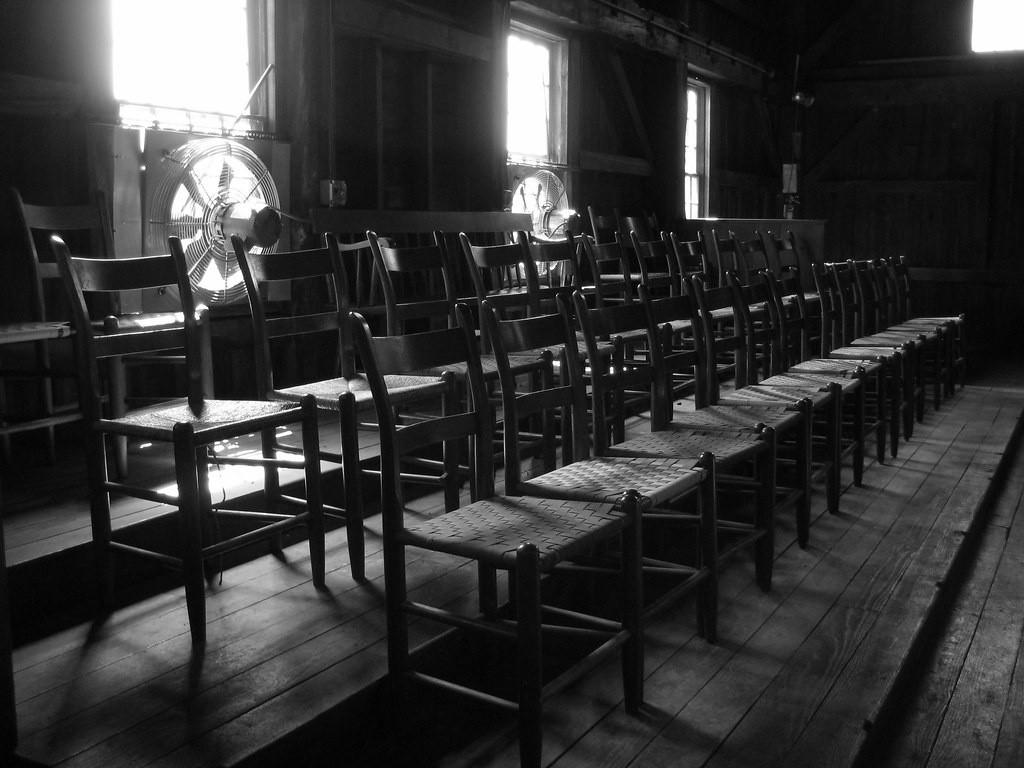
[505,170,575,280]
[148,138,282,310]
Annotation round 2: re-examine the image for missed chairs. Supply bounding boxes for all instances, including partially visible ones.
[5,186,965,767]
